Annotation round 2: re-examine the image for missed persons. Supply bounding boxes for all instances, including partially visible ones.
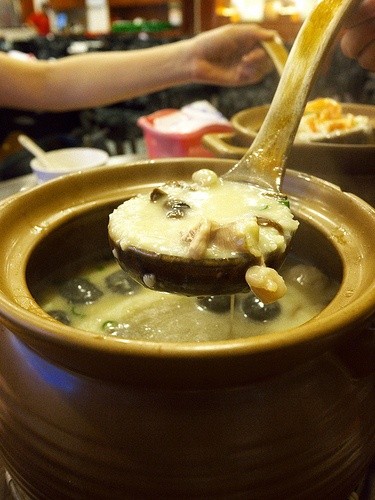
[340,0,375,71]
[0,23,278,111]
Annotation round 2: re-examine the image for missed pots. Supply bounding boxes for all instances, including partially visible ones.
[200,100,375,205]
[1,157,375,500]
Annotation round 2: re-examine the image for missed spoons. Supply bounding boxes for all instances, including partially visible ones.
[15,132,73,173]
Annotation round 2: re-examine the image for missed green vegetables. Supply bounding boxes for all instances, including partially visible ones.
[55,196,290,329]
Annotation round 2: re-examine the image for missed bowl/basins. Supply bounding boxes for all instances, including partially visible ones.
[137,107,229,158]
[31,147,110,183]
